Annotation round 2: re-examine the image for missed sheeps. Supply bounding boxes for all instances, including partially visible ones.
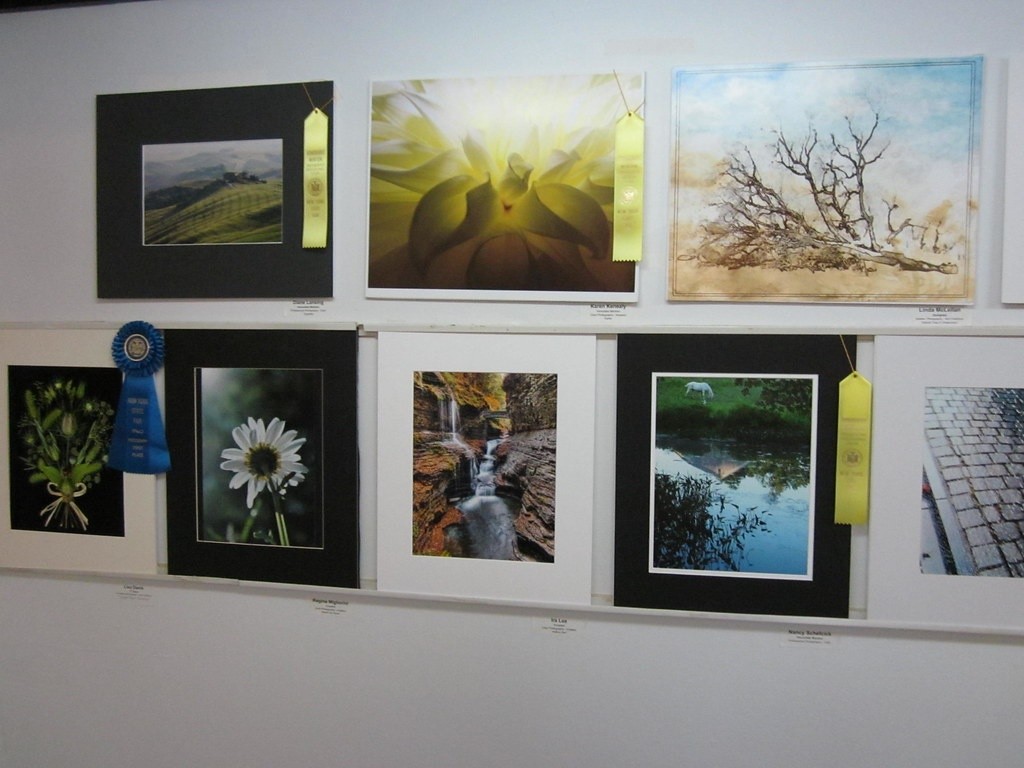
[684,381,713,402]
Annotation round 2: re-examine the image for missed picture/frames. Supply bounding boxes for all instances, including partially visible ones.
[375,330,596,605]
[165,328,359,588]
[0,330,158,574]
[96,79,333,300]
[668,55,983,307]
[612,332,857,620]
